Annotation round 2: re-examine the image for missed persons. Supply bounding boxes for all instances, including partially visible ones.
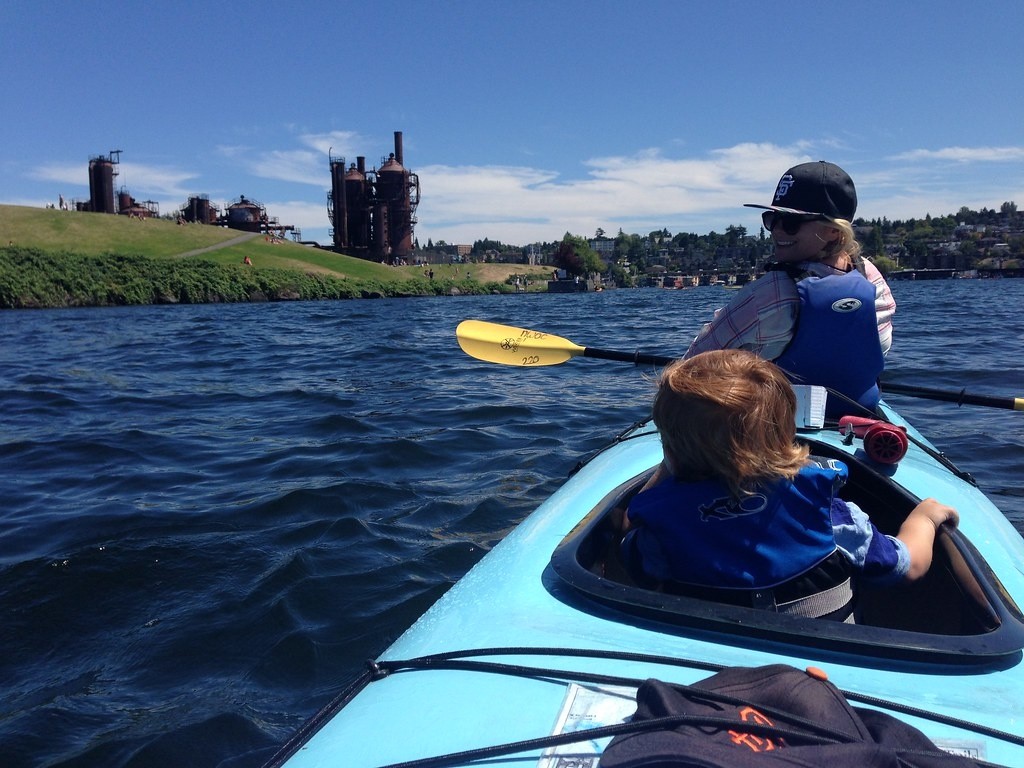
[523,274,529,292]
[45,192,69,211]
[389,257,470,281]
[515,275,520,293]
[129,211,134,218]
[138,214,145,220]
[622,348,961,629]
[177,217,186,226]
[551,272,556,281]
[682,160,896,425]
[244,256,251,266]
[265,236,284,246]
[574,275,579,290]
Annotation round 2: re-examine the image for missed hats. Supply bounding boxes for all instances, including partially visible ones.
[742,160,857,226]
[636,663,874,753]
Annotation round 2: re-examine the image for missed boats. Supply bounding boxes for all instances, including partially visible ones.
[256,393,1022,766]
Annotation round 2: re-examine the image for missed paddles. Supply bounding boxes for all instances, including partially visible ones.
[456,319,1024,413]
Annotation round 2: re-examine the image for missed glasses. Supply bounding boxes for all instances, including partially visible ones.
[762,210,830,235]
